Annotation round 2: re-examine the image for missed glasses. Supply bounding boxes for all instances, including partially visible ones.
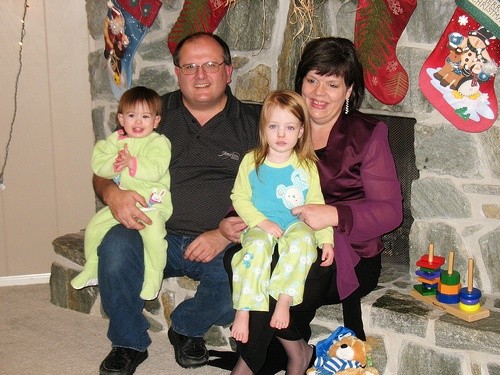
[178,61,225,75]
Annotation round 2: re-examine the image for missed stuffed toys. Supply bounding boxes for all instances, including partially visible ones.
[305,336,380,375]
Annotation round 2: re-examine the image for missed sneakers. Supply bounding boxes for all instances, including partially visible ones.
[98,347,148,375]
[167,325,209,369]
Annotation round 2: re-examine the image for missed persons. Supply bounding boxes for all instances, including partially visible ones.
[230,89,335,343]
[70,86,173,300]
[92,31,260,375]
[219,37,403,375]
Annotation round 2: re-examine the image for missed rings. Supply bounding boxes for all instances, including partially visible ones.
[237,239,240,245]
